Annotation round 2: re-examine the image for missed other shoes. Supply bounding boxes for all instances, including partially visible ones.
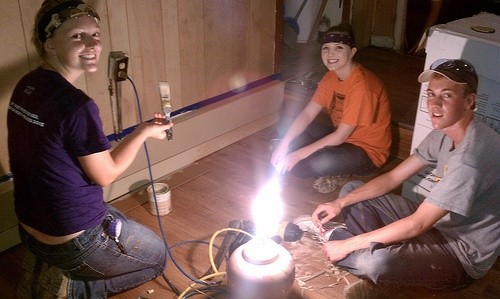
[268,139,282,151]
[13,250,39,298]
[343,280,407,299]
[292,215,348,242]
[313,173,351,193]
[37,266,64,299]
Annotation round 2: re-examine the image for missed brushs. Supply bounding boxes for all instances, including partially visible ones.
[157,81,174,141]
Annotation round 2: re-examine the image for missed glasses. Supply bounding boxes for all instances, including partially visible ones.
[44,5,100,38]
[429,59,474,75]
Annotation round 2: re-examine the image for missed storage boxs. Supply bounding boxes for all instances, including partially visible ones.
[401,13,500,226]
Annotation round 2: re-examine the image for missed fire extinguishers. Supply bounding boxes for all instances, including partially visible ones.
[317,13,331,43]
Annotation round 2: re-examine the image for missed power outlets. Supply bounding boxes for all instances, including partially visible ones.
[109,55,129,82]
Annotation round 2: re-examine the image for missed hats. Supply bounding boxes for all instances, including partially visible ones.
[418,57,478,94]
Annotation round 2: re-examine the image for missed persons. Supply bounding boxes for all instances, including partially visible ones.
[292,56,500,299]
[6,0,174,299]
[271,23,395,194]
